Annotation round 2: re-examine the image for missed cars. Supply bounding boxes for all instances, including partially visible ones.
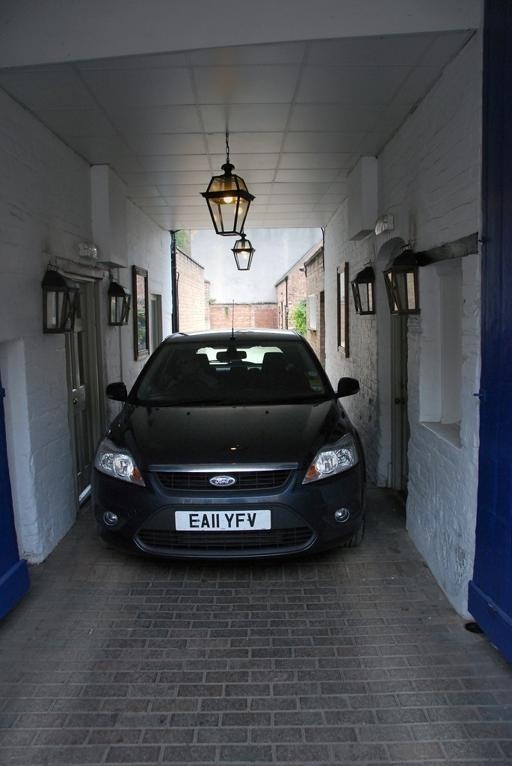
[90,324,369,568]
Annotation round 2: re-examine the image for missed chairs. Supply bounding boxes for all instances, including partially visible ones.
[262,351,290,383]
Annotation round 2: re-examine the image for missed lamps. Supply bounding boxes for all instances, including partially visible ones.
[380,249,420,314]
[108,282,129,326]
[41,270,72,333]
[354,267,376,315]
[199,134,256,270]
